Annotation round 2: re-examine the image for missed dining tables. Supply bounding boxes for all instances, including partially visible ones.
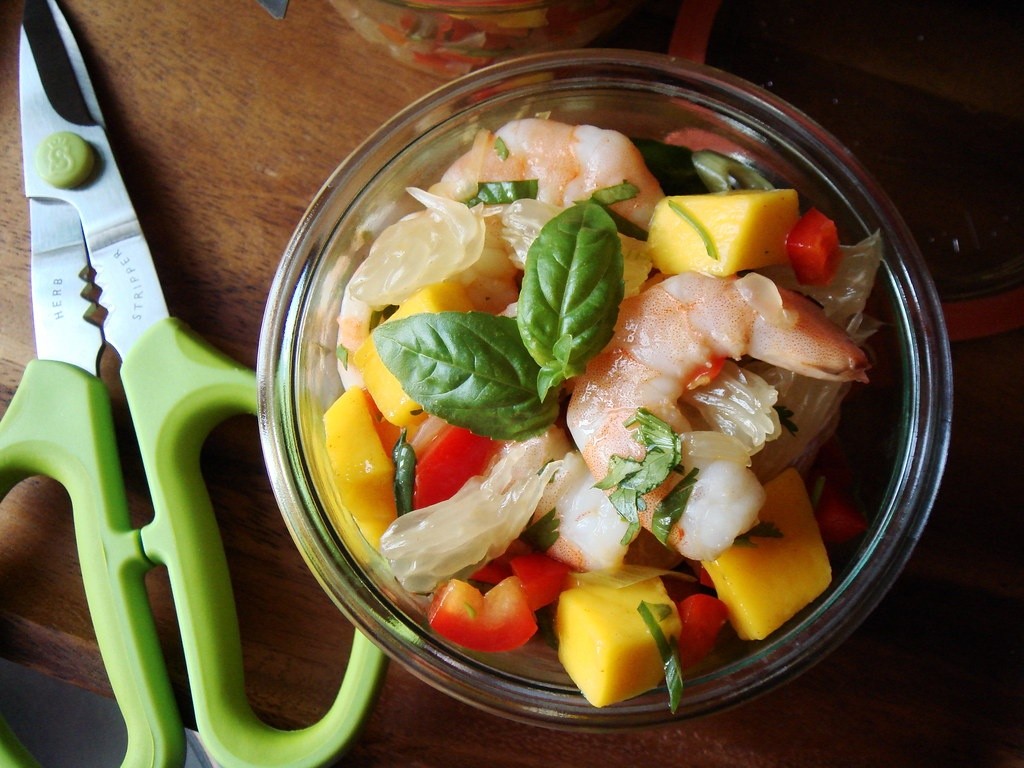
[0,0,1022,768]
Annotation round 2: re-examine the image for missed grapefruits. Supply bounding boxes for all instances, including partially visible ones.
[673,232,884,479]
[352,187,489,304]
[378,438,570,593]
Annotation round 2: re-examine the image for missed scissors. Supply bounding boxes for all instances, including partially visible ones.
[0,0,388,768]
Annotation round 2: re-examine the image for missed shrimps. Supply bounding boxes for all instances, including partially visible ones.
[337,115,871,578]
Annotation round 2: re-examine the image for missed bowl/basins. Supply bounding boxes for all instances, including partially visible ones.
[256,47,954,733]
